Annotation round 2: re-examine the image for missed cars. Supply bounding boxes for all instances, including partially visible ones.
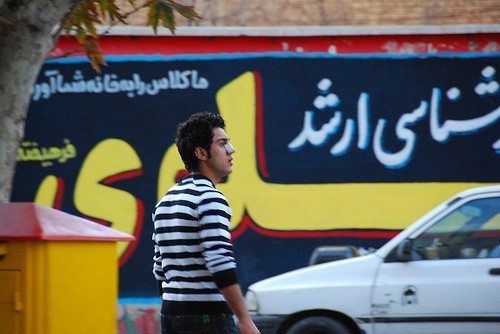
[233,186,500,333]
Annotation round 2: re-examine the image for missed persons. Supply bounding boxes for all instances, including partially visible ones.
[151,112,261,334]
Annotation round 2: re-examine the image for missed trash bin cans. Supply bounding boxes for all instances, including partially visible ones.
[0,201,137,334]
